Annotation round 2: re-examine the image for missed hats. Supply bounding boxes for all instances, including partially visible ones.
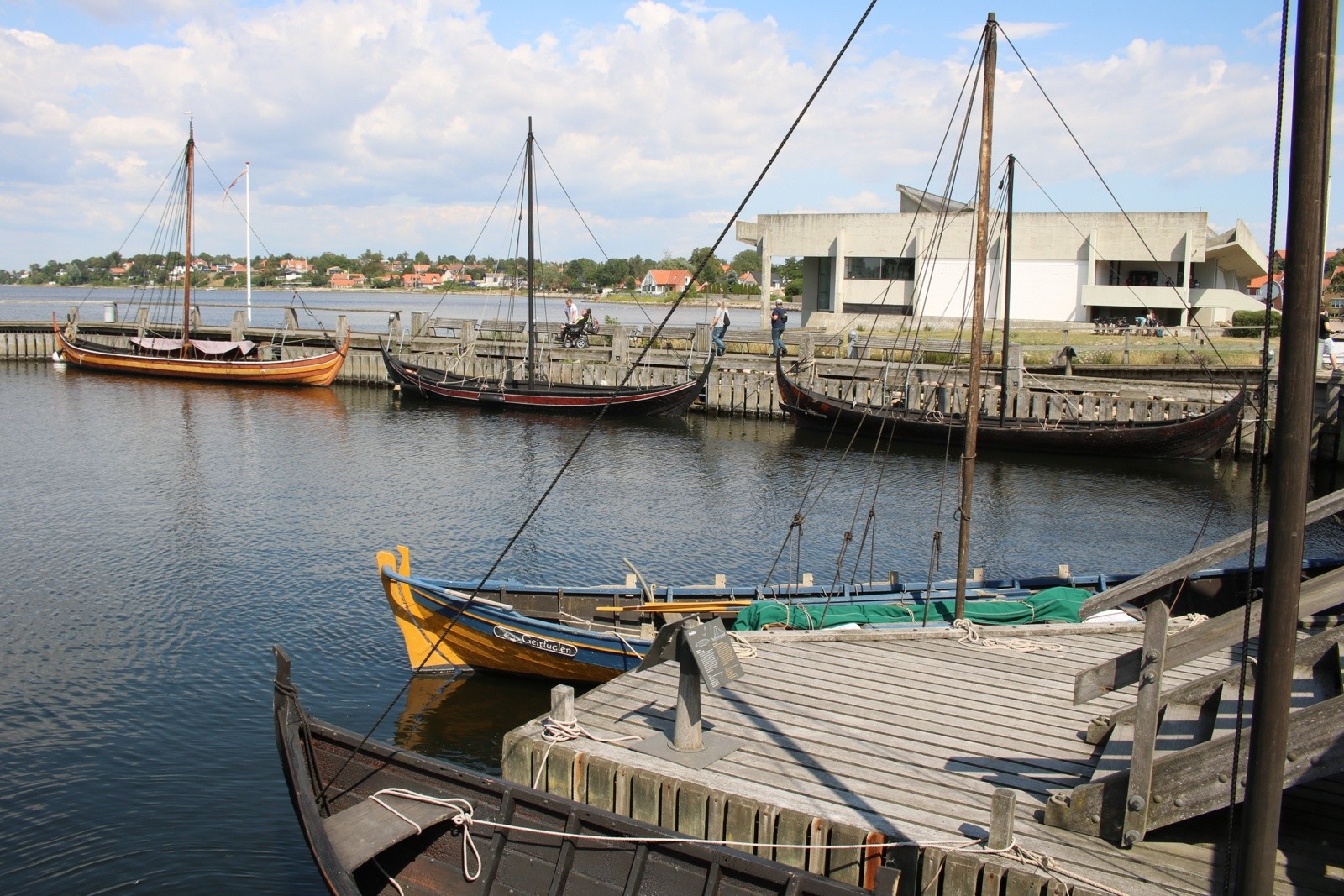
[775,299,782,304]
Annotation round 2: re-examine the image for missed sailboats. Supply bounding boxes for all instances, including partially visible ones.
[53,114,352,390]
[374,115,716,417]
[271,1,1344,895]
[776,11,1245,462]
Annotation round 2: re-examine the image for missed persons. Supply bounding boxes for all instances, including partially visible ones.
[709,301,728,356]
[555,308,588,342]
[564,299,578,324]
[1146,309,1156,340]
[1134,316,1146,328]
[1318,305,1344,374]
[1125,275,1200,288]
[769,299,787,358]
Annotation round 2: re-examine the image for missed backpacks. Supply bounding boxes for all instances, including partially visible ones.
[781,313,787,323]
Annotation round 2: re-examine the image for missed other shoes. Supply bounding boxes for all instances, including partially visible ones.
[722,346,727,355]
[782,350,787,357]
[1333,369,1344,374]
[769,353,776,357]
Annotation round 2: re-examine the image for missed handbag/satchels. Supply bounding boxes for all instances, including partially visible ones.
[584,315,592,332]
[591,317,599,335]
[723,312,730,326]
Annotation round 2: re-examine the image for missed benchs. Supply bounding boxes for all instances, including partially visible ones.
[320,786,475,874]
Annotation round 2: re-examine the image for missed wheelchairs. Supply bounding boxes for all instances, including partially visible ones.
[555,309,591,349]
